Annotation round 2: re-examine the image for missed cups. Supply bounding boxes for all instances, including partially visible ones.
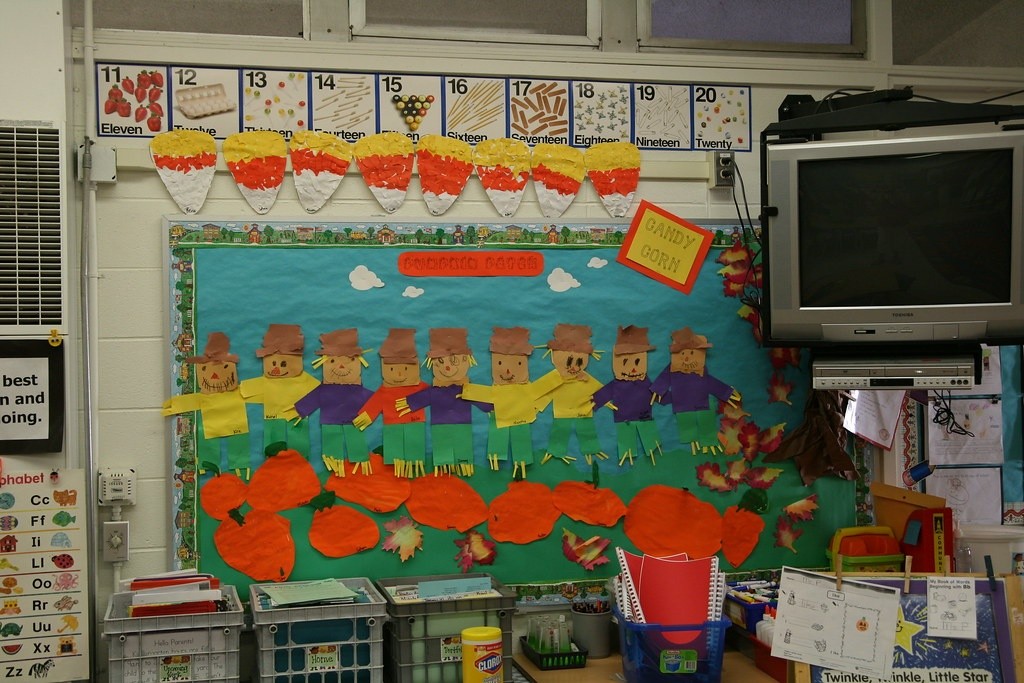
[571,605,612,658]
[957,547,971,573]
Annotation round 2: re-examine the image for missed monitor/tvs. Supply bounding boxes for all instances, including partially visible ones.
[767,129,1024,341]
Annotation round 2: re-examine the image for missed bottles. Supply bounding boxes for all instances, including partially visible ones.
[528,616,569,666]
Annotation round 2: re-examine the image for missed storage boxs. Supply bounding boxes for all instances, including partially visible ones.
[959,525,1024,578]
[727,583,787,683]
[614,606,731,683]
[250,577,389,683]
[104,586,243,683]
[376,573,517,683]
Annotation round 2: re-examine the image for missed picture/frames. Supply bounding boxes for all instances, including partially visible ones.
[922,467,1004,526]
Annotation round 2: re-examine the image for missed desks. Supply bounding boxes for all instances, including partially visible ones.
[510,652,781,683]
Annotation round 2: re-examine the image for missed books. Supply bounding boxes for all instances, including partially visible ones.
[612,546,725,674]
